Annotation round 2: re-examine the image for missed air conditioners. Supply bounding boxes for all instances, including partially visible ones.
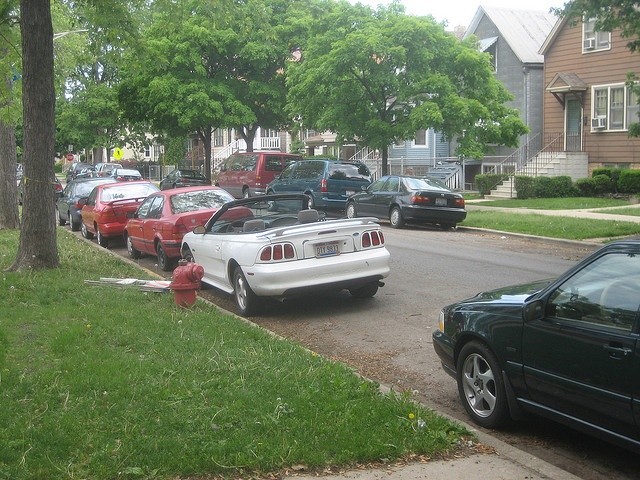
[583,38,595,50]
[591,116,605,129]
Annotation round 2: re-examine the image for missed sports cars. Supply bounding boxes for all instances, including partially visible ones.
[181,194,390,316]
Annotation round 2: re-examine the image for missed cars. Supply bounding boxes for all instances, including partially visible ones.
[97,163,124,178]
[16,163,24,179]
[344,176,467,231]
[79,180,163,249]
[432,238,639,457]
[111,168,144,182]
[56,178,118,231]
[124,187,256,272]
[66,163,96,183]
[160,170,212,191]
[17,176,63,209]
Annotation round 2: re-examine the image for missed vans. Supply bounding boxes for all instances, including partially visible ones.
[265,158,375,217]
[216,152,305,199]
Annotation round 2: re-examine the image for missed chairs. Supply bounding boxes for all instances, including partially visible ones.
[294,208,318,226]
[243,219,265,233]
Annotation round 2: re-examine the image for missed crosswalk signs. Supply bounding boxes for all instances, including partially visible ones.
[112,147,124,160]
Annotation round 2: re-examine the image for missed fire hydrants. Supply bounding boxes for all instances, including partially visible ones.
[169,259,204,310]
